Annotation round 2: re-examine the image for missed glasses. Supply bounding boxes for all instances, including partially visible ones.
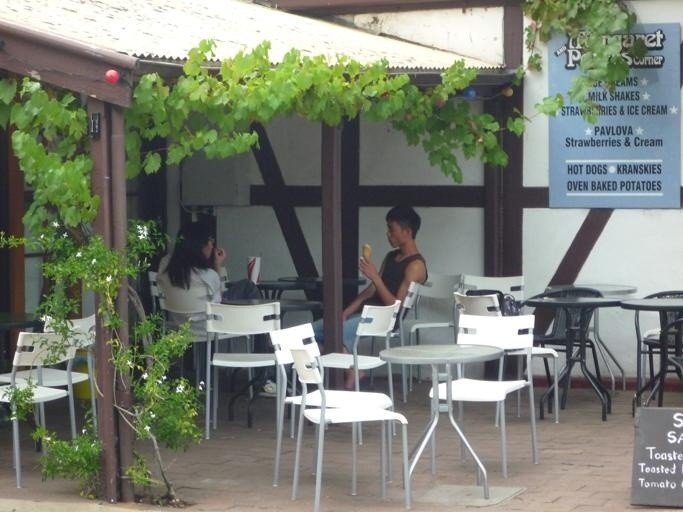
[208,238,215,244]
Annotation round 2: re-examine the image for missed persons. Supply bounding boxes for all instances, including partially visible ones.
[157,229,278,395]
[283,202,428,387]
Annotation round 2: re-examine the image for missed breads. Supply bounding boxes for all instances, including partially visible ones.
[362,244,371,257]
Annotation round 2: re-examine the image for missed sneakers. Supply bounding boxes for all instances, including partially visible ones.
[257,379,289,398]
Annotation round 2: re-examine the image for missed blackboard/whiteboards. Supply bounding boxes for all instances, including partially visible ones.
[631,407,683,507]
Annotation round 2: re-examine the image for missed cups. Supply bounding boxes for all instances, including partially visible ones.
[247,255,263,285]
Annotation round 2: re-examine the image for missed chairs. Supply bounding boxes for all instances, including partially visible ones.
[128,268,681,511]
[1,312,96,488]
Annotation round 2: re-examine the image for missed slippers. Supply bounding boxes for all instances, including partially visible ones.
[344,375,370,391]
